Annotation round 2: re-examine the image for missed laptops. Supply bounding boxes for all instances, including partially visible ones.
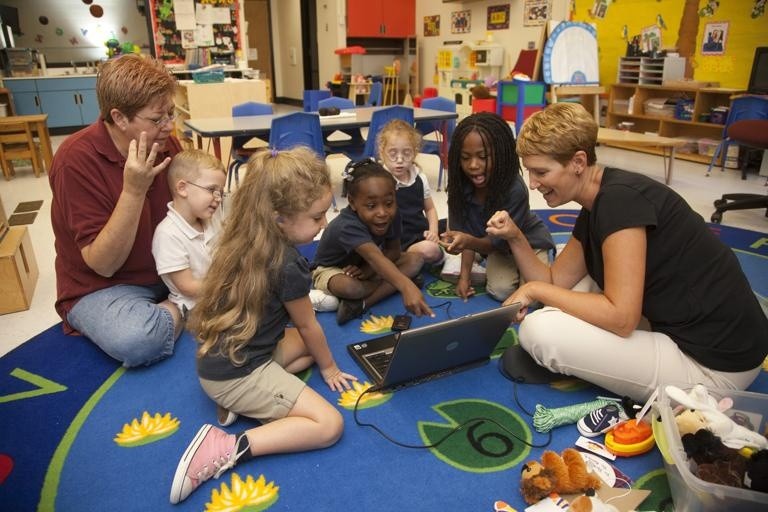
[346,301,522,394]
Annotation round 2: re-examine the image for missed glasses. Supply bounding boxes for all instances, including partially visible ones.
[188,181,228,198]
[137,112,177,130]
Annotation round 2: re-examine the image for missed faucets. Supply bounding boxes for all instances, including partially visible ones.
[70,60,78,73]
[86,59,98,72]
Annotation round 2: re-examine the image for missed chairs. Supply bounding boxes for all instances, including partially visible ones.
[706,96,768,177]
[227,81,456,207]
[0,122,44,181]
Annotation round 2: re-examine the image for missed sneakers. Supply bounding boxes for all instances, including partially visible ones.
[577,404,621,437]
[170,424,236,504]
[440,254,487,286]
[338,299,363,326]
[311,290,338,312]
[218,404,237,427]
[413,275,423,288]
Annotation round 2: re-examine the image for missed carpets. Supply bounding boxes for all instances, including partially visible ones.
[0,209,767,511]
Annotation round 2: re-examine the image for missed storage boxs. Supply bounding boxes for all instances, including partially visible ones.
[698,136,719,157]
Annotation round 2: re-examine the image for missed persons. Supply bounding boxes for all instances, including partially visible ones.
[48,52,188,369]
[310,157,436,326]
[438,110,555,309]
[376,119,446,272]
[150,150,340,322]
[170,146,358,504]
[485,102,768,407]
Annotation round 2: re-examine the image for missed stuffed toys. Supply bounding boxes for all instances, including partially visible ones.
[664,384,768,494]
[519,450,600,504]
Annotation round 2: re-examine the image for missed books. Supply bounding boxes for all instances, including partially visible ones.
[645,102,675,118]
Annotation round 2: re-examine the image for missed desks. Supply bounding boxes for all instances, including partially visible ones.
[183,105,458,193]
[597,128,687,184]
[0,115,53,173]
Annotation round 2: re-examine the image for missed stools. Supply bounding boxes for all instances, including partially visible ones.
[711,120,768,223]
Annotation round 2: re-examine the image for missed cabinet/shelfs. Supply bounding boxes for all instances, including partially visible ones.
[176,82,266,169]
[35,77,99,128]
[347,0,416,38]
[604,83,747,167]
[617,57,686,85]
[3,78,43,128]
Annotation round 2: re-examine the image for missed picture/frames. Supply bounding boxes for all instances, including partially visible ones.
[523,0,553,26]
[487,4,510,30]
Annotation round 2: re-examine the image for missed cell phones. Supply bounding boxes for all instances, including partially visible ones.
[391,315,412,332]
